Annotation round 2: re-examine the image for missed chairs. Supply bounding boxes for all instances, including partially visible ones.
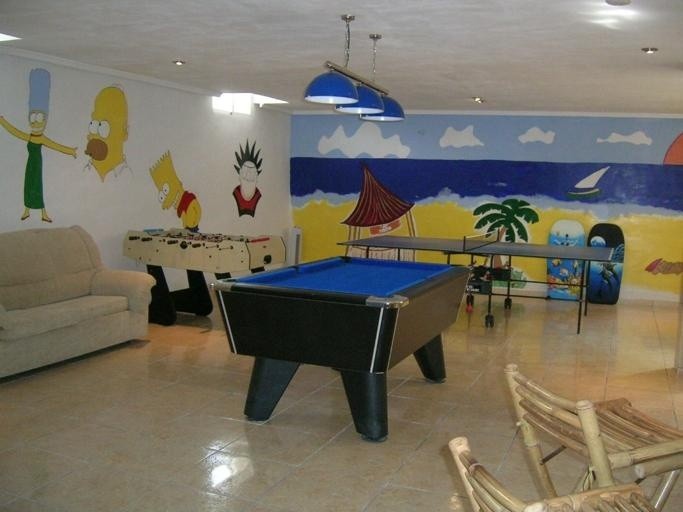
[447,430,657,512]
[503,362,682,512]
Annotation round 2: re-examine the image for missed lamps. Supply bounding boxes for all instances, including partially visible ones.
[303,14,405,122]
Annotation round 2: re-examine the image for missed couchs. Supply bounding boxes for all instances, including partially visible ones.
[0,223,158,379]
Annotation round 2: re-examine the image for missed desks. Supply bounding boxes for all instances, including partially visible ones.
[212,254,470,443]
[335,229,613,334]
[122,227,287,325]
